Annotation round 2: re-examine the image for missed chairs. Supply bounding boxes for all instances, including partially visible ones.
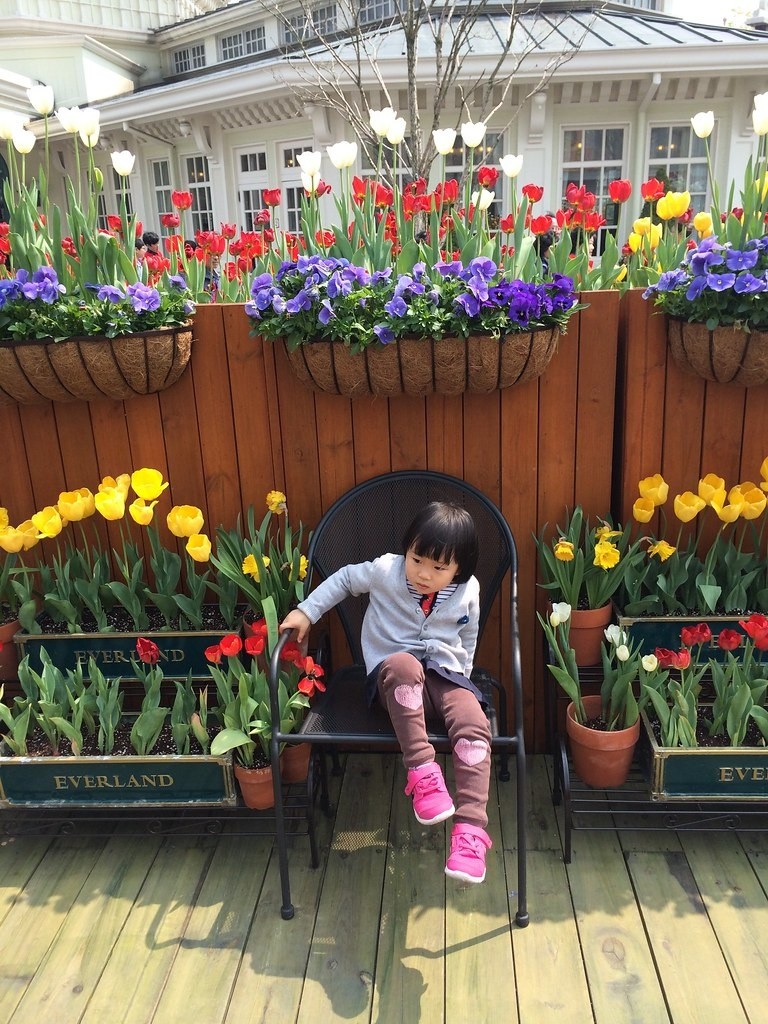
[266,470,529,926]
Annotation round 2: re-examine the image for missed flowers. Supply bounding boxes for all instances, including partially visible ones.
[0,266,197,343]
[535,600,670,732]
[0,467,313,634]
[0,508,24,622]
[204,617,326,769]
[620,458,768,617]
[640,231,768,335]
[532,503,676,610]
[0,637,229,756]
[643,614,768,748]
[247,620,304,744]
[245,254,591,358]
[209,490,315,623]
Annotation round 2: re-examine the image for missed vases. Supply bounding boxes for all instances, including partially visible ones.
[614,608,768,663]
[283,324,560,401]
[0,290,618,753]
[637,703,768,801]
[242,609,309,678]
[566,696,640,789]
[231,742,284,810]
[0,617,22,684]
[12,603,251,682]
[0,317,194,407]
[547,592,613,667]
[666,314,768,388]
[617,289,768,607]
[0,711,237,808]
[279,741,310,783]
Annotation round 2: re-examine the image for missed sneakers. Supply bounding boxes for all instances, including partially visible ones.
[404,762,454,826]
[444,815,493,882]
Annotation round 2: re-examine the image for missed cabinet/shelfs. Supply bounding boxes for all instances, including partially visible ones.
[539,619,768,864]
[0,602,340,920]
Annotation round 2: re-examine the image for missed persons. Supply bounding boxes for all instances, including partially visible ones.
[125,239,148,291]
[179,240,223,303]
[534,212,595,291]
[144,231,169,289]
[279,503,493,885]
